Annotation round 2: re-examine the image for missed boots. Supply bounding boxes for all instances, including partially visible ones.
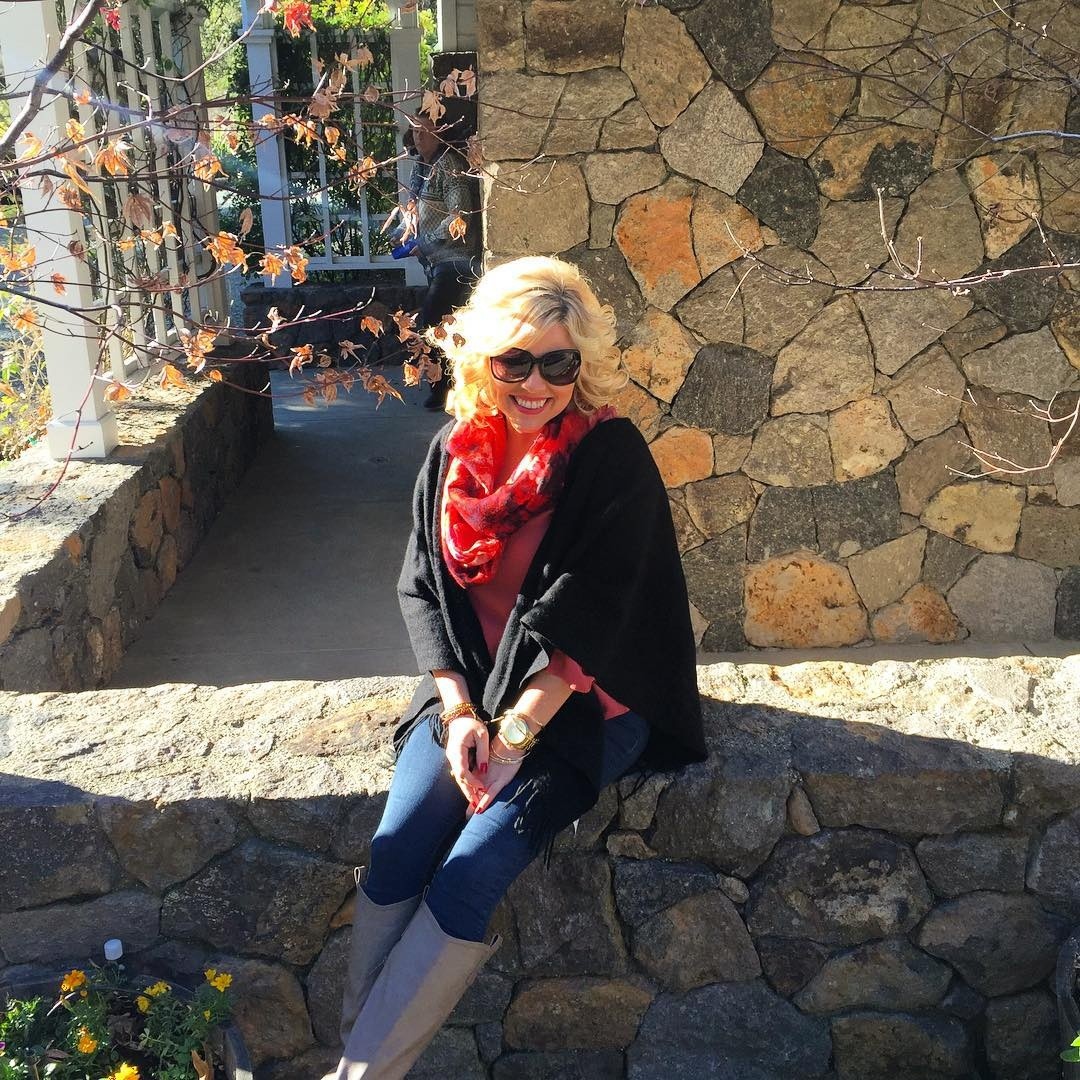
[321,886,504,1080]
[340,865,422,1049]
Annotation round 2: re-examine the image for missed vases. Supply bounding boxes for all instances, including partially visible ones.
[0,971,253,1080]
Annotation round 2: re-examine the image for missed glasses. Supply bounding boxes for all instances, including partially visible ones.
[490,348,582,387]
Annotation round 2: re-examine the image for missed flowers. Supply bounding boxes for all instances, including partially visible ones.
[0,958,239,1080]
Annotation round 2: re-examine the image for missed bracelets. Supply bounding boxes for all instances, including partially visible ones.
[488,710,543,764]
[439,703,477,724]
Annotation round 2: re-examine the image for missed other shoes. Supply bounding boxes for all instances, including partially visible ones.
[423,375,451,409]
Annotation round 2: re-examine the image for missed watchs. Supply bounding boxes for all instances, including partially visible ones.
[499,716,534,750]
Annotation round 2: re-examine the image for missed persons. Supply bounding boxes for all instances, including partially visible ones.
[390,108,473,407]
[321,255,709,1080]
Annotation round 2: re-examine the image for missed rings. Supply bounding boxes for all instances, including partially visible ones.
[450,771,453,776]
